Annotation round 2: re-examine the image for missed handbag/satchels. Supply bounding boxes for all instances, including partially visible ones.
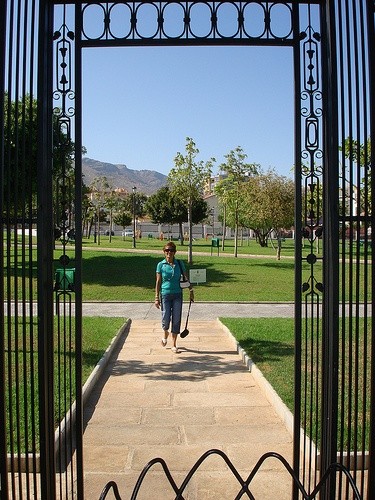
[178,260,190,289]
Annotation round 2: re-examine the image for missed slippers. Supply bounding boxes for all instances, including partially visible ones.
[171,347,181,353]
[161,332,169,346]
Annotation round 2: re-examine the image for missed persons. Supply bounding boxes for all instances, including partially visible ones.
[155,241,194,353]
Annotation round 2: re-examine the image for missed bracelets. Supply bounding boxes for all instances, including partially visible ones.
[190,289,193,292]
[155,296,159,300]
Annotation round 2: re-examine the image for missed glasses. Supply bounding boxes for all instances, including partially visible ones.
[164,249,175,252]
